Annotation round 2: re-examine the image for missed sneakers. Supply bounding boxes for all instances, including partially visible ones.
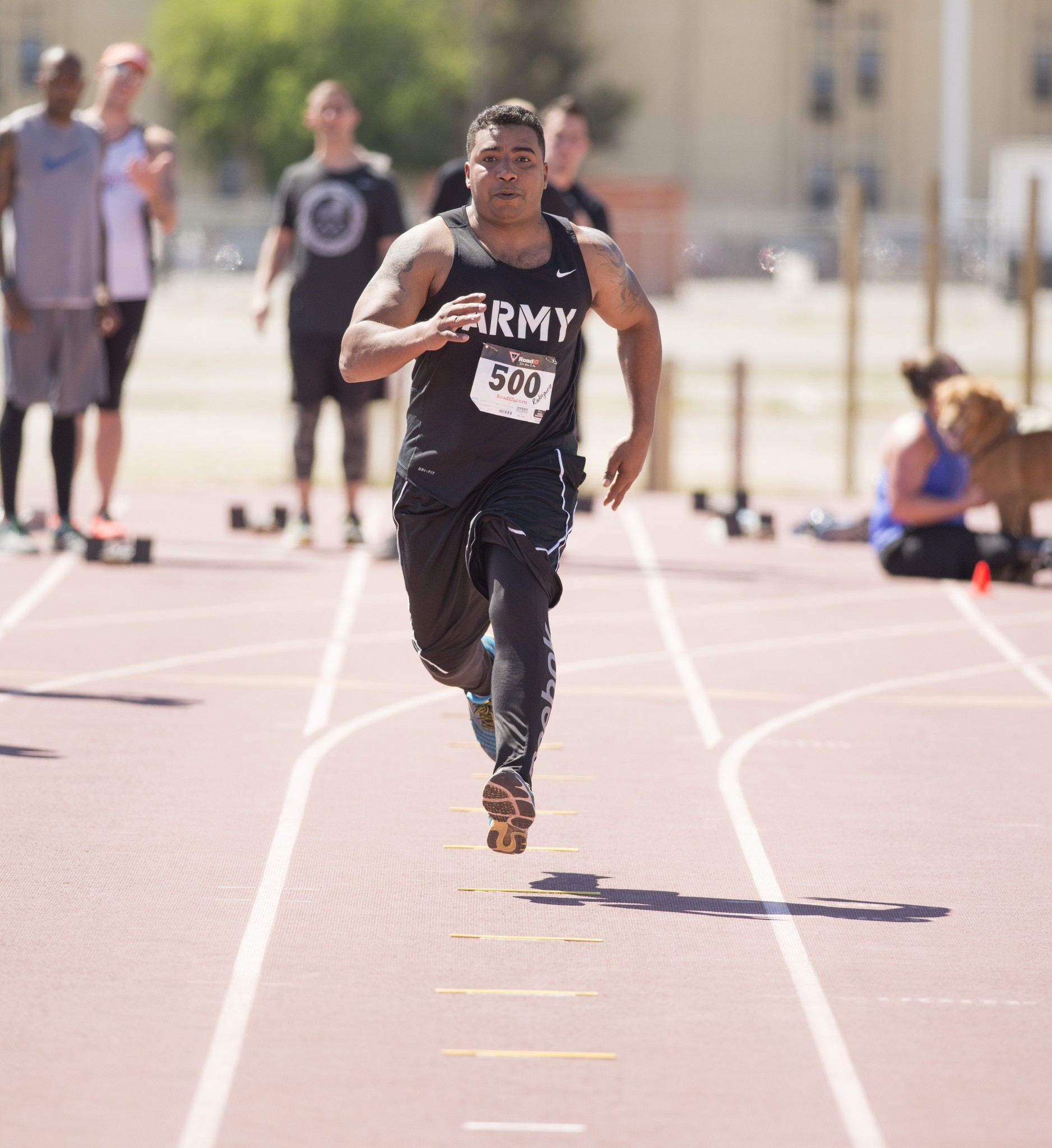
[481,766,536,855]
[464,635,497,762]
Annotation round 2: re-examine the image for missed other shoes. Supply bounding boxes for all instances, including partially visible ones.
[342,514,364,544]
[1,518,37,553]
[52,526,94,557]
[87,516,124,538]
[43,514,83,533]
[296,518,315,545]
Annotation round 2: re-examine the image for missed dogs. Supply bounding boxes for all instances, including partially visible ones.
[932,373,1051,540]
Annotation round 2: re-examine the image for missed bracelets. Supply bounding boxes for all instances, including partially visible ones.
[1,278,14,291]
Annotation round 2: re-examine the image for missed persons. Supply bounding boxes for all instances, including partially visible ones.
[868,351,1052,584]
[375,94,613,559]
[252,77,407,549]
[0,42,178,549]
[339,104,662,856]
